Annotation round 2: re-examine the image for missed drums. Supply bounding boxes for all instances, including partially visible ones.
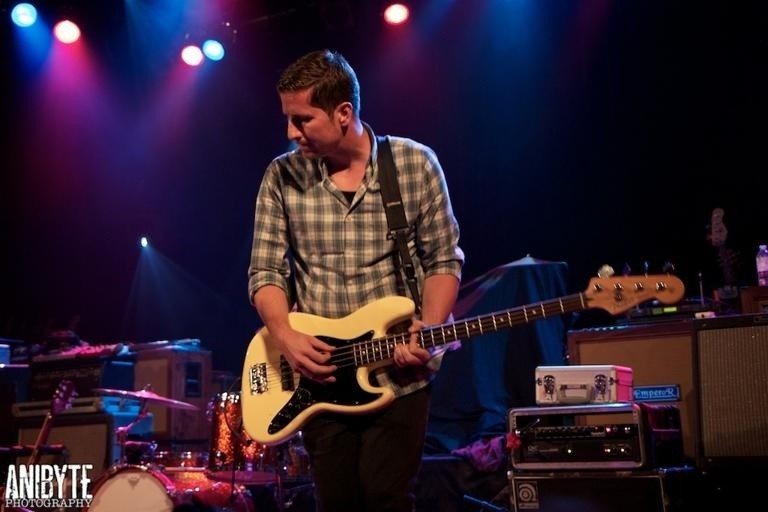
[142,448,209,471]
[206,391,302,486]
[78,459,256,512]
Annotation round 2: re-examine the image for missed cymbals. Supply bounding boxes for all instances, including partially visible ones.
[85,386,199,413]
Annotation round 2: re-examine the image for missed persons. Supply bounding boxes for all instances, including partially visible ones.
[249,50,466,512]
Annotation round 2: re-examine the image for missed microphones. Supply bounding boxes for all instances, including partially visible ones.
[696,272,708,312]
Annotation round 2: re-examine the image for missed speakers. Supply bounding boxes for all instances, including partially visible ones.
[510,473,667,511]
[133,347,213,443]
[15,411,115,485]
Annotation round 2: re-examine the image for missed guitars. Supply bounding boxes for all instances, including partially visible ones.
[240,261,684,446]
[2,379,78,511]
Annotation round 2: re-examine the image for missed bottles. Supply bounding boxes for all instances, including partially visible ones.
[756,244,768,286]
